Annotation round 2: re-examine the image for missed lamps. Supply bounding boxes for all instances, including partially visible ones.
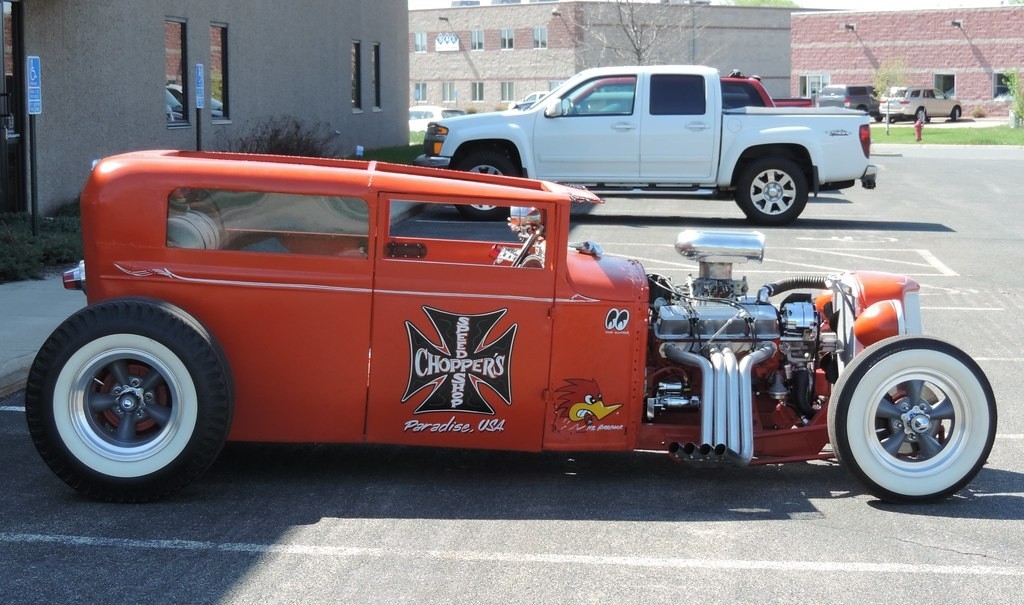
[944,20,964,28]
[840,23,855,32]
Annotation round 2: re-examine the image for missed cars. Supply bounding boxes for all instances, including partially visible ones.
[508,90,552,110]
[23,146,998,503]
[408,105,467,136]
[162,80,224,126]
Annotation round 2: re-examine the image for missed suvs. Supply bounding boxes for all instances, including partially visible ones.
[878,86,962,124]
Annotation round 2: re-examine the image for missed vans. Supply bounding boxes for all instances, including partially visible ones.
[818,83,884,121]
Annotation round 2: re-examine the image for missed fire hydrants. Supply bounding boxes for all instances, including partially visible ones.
[913,120,923,142]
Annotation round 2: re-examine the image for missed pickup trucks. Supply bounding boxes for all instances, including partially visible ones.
[564,70,815,113]
[412,65,878,228]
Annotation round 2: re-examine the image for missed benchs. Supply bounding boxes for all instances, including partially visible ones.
[168,209,218,250]
